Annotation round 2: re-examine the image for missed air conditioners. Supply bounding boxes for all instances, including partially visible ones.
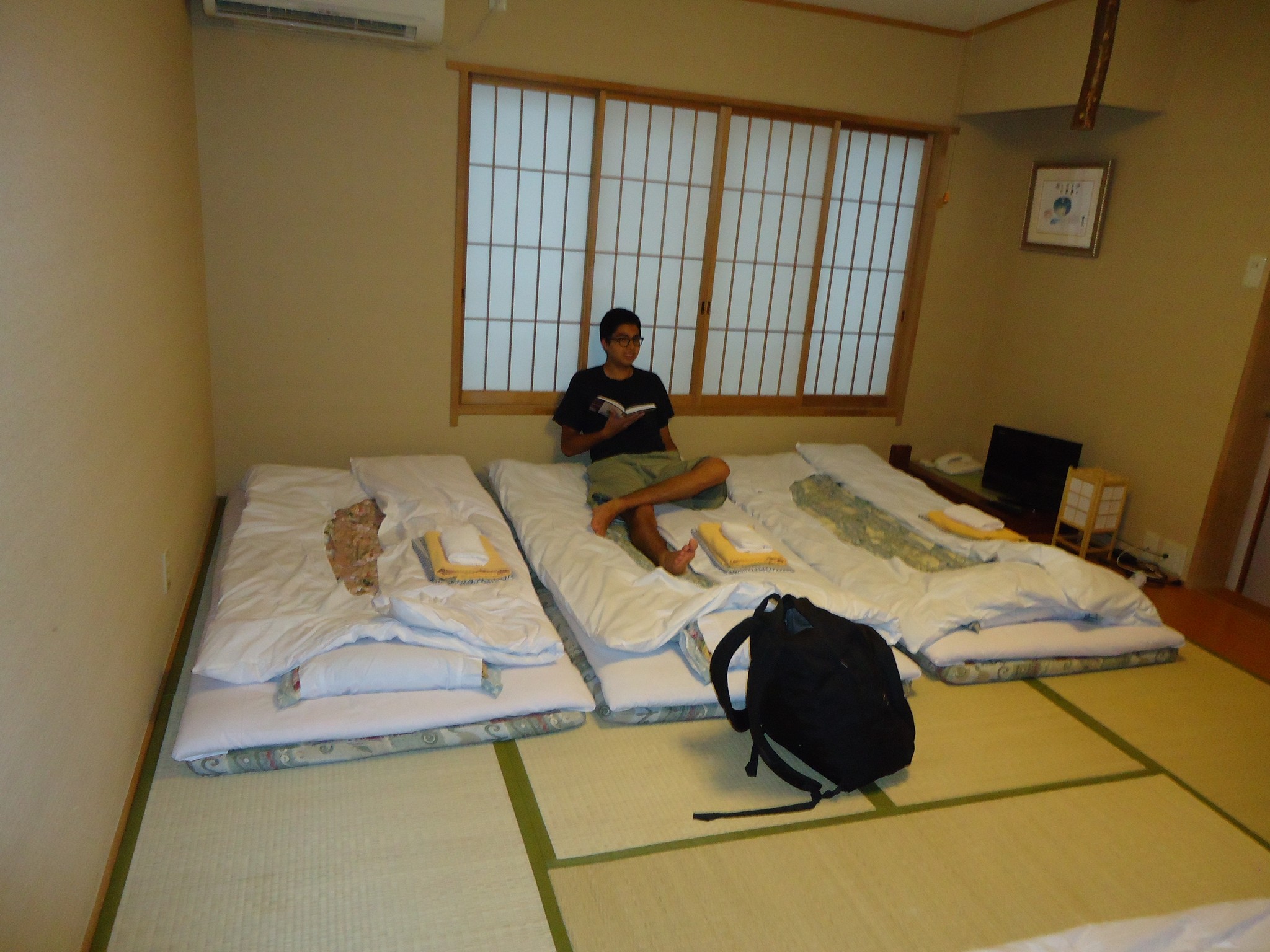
[199,0,445,49]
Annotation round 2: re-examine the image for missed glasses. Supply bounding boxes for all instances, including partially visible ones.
[609,337,644,348]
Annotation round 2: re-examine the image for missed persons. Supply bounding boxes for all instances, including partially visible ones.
[554,307,731,576]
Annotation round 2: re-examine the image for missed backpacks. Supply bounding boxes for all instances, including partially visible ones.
[692,593,916,823]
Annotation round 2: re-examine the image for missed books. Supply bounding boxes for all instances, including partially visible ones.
[589,395,656,421]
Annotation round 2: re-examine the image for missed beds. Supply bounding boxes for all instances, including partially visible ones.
[180,437,1185,793]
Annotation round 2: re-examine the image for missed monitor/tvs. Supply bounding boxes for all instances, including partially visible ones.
[982,424,1083,518]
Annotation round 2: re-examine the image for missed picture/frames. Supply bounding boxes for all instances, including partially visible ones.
[1020,156,1115,259]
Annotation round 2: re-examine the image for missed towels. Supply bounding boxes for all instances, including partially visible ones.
[926,503,1029,542]
[411,523,518,585]
[690,521,795,575]
